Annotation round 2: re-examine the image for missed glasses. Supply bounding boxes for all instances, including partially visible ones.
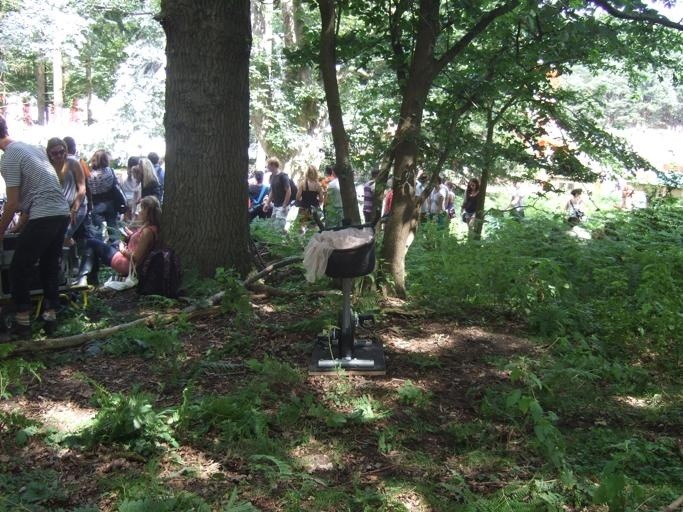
[50,148,65,156]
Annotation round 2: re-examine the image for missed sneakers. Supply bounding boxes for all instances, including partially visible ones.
[0,322,32,342]
[40,318,57,336]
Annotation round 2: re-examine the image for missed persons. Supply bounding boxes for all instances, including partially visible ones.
[68,194,159,292]
[566,188,584,224]
[459,177,480,235]
[504,181,525,217]
[0,118,68,339]
[248,156,344,230]
[363,169,457,234]
[47,137,164,243]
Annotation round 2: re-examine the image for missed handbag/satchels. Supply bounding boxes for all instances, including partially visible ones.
[111,180,128,214]
[288,179,297,199]
[105,274,139,291]
[136,251,179,294]
